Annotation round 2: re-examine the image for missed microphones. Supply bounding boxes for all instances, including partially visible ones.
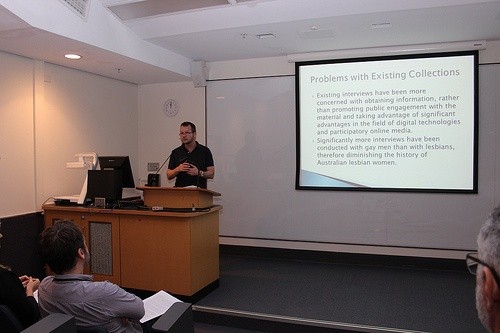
[186,158,200,188]
[149,151,175,186]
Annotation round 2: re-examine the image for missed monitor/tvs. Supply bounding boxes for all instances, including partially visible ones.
[97,155,135,188]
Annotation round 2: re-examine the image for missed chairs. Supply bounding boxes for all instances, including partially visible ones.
[0,305,23,332]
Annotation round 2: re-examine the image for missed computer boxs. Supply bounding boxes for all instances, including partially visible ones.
[87,169,122,204]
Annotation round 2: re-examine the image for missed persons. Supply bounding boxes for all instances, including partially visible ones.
[38,219,163,333]
[466,205,500,333]
[167,122,214,189]
[0,222,41,333]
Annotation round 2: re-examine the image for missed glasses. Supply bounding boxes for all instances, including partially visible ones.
[466,251,500,291]
[179,131,194,136]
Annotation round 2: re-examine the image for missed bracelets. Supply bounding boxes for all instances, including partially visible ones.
[200,170,203,177]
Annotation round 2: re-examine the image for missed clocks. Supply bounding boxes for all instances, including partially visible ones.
[163,98,180,117]
